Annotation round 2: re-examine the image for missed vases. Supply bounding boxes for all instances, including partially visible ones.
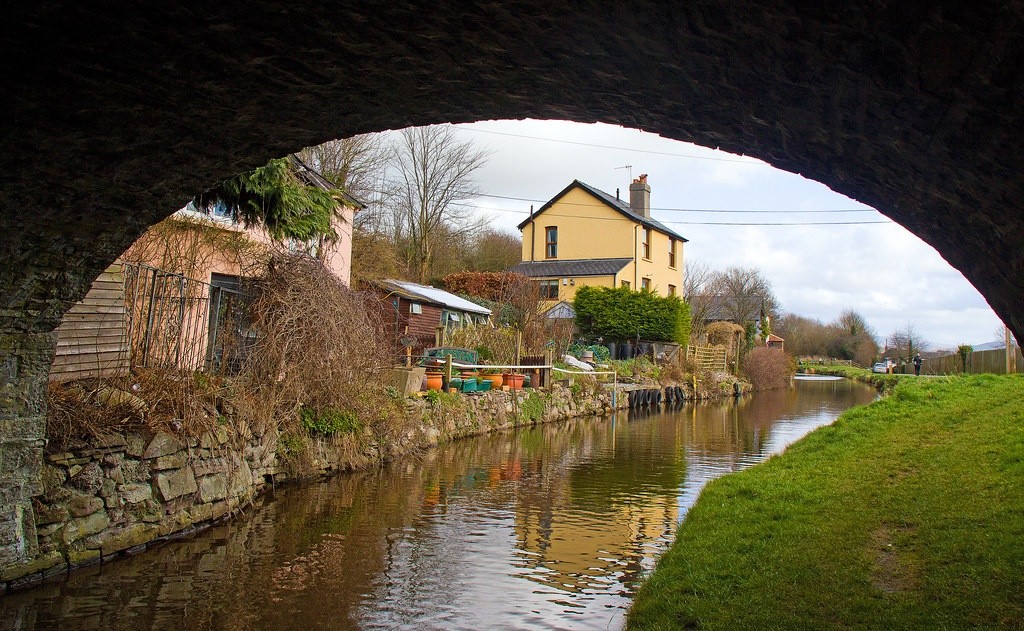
[425,369,540,395]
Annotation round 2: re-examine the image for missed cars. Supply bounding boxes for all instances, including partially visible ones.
[872,363,887,375]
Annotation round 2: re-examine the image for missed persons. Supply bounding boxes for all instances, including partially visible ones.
[914,353,922,376]
